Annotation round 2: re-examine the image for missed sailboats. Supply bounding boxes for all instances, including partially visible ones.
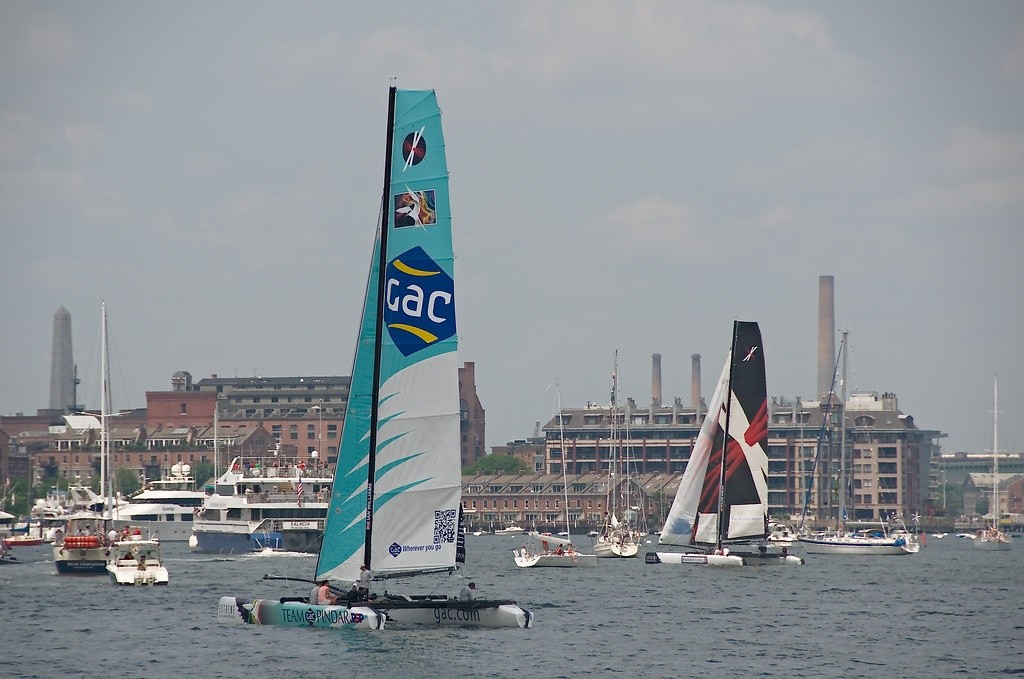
[513,350,648,567]
[217,75,534,630]
[973,369,1014,550]
[644,315,805,569]
[767,326,920,555]
[52,299,160,576]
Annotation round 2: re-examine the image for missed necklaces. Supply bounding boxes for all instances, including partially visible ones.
[318,580,336,604]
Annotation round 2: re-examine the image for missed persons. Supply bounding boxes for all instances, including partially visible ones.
[521,545,526,557]
[356,564,374,602]
[108,525,141,541]
[460,582,475,601]
[147,551,153,558]
[77,529,83,536]
[56,529,62,533]
[782,547,787,557]
[234,461,329,503]
[715,547,721,555]
[705,546,713,554]
[512,548,520,558]
[557,544,564,555]
[348,583,358,600]
[310,581,321,604]
[722,547,729,556]
[124,553,134,560]
[568,547,577,562]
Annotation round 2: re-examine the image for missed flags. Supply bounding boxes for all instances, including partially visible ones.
[298,478,304,507]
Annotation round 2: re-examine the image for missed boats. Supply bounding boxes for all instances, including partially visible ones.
[104,522,169,584]
[0,398,335,554]
[495,521,524,535]
